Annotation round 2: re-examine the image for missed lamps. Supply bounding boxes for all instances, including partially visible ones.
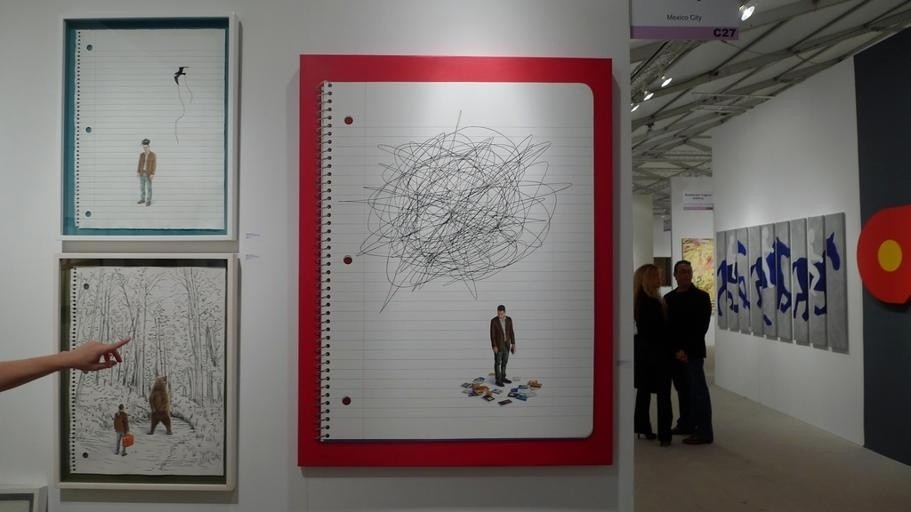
[736,0,755,21]
[631,64,674,111]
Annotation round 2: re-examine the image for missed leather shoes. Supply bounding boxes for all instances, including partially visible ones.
[496,382,504,386]
[682,434,713,445]
[503,380,512,383]
[672,425,693,435]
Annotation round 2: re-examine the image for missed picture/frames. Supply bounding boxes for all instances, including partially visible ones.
[299,54,615,467]
[56,11,237,245]
[0,485,48,512]
[58,258,239,493]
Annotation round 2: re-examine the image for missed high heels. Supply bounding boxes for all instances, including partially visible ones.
[661,440,670,447]
[638,430,657,440]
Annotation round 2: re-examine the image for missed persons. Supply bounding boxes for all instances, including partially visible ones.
[654,259,715,446]
[0,336,132,390]
[490,304,518,387]
[136,138,158,207]
[112,403,130,456]
[633,262,676,448]
